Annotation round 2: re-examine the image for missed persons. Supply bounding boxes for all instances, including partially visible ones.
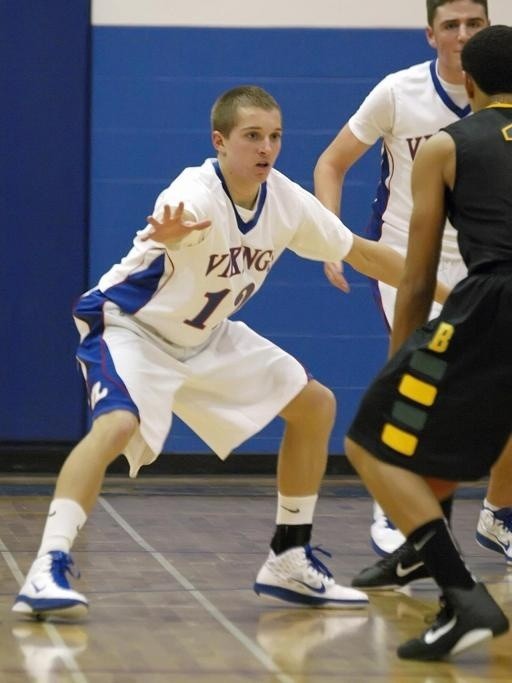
[313,0,512,566]
[345,25,512,661]
[12,86,450,621]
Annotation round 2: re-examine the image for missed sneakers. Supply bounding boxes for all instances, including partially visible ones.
[352,500,512,591]
[12,550,89,622]
[396,581,510,664]
[253,543,370,609]
